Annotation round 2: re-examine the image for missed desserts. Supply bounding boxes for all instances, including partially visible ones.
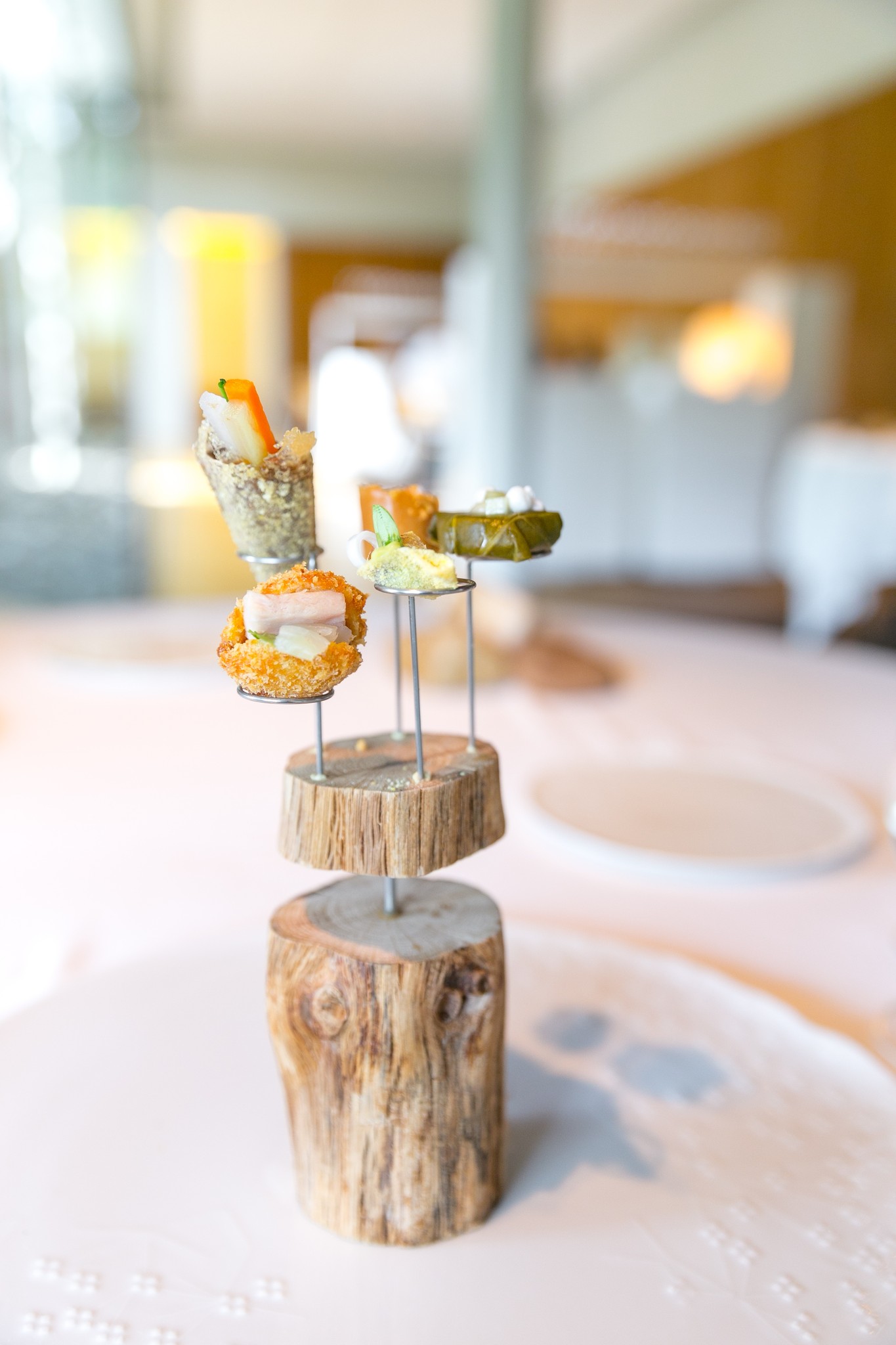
[196,376,319,584]
[434,487,561,563]
[360,504,460,601]
[219,568,367,698]
[360,483,439,560]
